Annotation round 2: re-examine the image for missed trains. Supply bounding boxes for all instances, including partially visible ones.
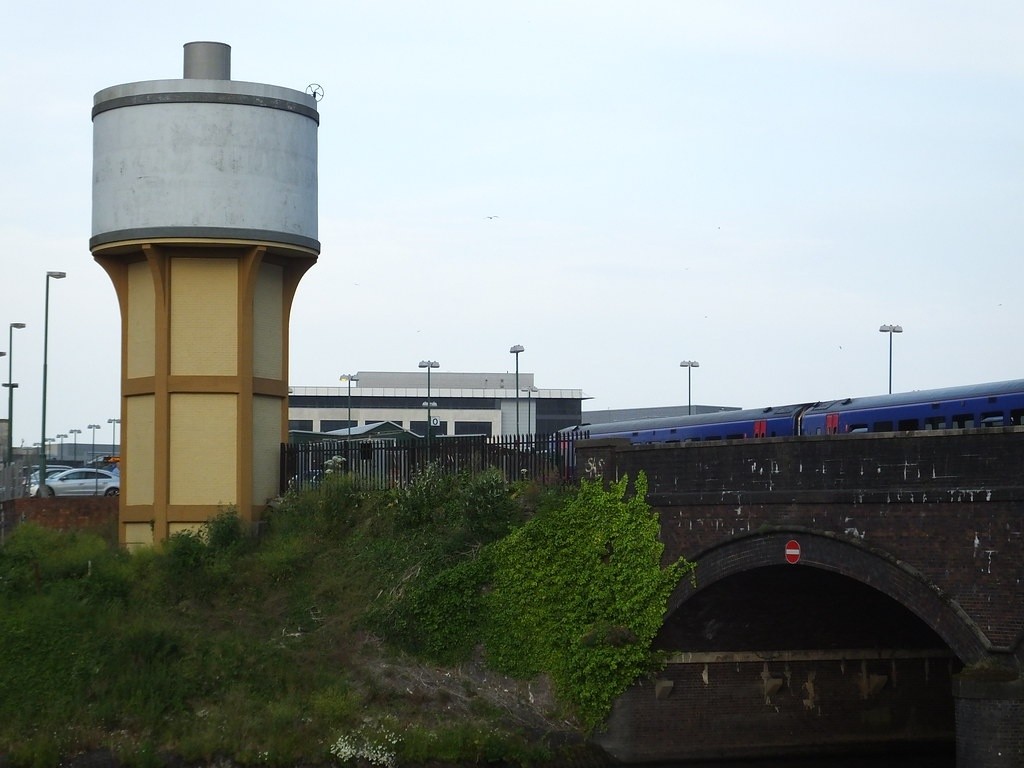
[547,378,1024,473]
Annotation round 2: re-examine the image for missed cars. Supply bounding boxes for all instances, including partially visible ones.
[18,463,119,498]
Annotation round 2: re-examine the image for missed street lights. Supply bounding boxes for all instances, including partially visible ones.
[679,360,699,415]
[509,344,526,440]
[417,359,440,435]
[878,323,903,394]
[339,373,360,440]
[520,386,538,434]
[38,271,67,499]
[3,322,27,464]
[31,418,120,467]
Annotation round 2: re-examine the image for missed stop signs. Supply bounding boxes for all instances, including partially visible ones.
[785,540,802,564]
